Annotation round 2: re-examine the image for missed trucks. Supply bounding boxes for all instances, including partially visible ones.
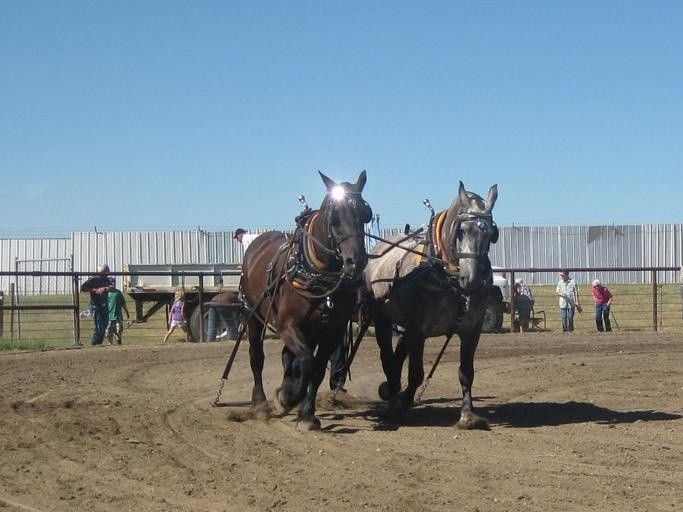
[127,264,511,334]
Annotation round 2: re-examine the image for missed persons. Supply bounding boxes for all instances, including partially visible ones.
[80,264,113,344]
[516,276,533,303]
[231,228,259,252]
[554,271,579,332]
[106,277,129,345]
[163,289,192,343]
[591,279,614,331]
[205,292,240,341]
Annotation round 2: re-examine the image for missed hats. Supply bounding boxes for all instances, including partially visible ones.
[232,229,247,238]
[98,264,109,272]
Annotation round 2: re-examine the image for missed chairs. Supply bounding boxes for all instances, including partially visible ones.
[530,309,546,331]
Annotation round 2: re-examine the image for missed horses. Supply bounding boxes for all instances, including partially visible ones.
[356,180,499,430]
[241,169,369,433]
